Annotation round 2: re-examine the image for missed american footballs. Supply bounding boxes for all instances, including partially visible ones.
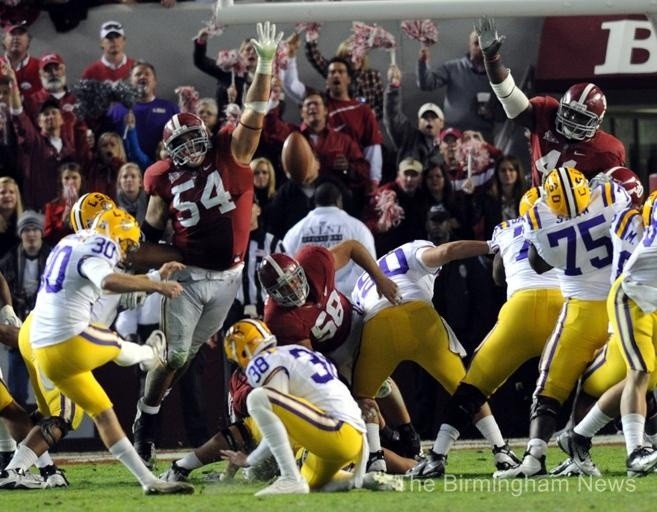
[280,132,313,184]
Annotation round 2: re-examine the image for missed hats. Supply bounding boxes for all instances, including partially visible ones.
[17,210,43,239]
[399,102,463,219]
[1,24,64,112]
[100,21,124,39]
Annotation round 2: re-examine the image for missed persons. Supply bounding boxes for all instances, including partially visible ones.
[0,2,657,500]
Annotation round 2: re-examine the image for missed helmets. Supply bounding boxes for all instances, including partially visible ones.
[163,112,213,167]
[555,82,608,141]
[258,254,310,307]
[70,192,141,263]
[519,166,645,217]
[223,318,277,373]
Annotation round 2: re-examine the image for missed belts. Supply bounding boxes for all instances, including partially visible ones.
[188,269,238,281]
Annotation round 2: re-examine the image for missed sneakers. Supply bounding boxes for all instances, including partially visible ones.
[139,329,167,371]
[347,422,447,492]
[253,476,310,496]
[0,451,69,490]
[492,427,657,480]
[132,411,194,495]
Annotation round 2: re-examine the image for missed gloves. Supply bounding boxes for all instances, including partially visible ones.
[473,16,506,64]
[251,21,285,75]
